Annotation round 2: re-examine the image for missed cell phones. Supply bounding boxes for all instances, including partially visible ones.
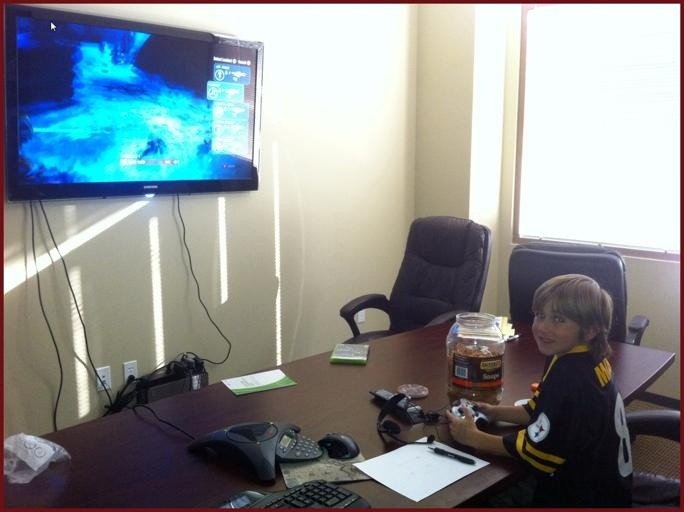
[369,388,426,424]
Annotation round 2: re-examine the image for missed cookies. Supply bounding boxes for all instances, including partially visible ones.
[397,384,429,399]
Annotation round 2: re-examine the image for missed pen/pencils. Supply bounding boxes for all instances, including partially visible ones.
[507,332,524,341]
[428,445,476,465]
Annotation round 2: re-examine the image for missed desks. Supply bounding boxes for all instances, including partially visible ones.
[5,311,677,509]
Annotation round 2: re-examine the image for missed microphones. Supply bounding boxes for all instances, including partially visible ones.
[389,432,435,444]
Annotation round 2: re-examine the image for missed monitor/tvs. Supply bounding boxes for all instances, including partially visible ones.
[5,4,263,200]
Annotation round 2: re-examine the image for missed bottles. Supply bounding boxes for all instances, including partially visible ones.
[445,312,506,403]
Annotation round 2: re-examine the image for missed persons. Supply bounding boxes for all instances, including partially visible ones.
[444,272,634,509]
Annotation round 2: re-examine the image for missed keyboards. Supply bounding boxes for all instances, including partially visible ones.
[240,479,371,509]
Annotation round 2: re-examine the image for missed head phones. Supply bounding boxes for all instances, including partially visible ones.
[377,394,409,434]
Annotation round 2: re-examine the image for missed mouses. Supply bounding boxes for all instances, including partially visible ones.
[319,433,359,459]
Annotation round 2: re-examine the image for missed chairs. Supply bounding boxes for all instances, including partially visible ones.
[339,215,491,346]
[508,240,648,347]
[624,409,680,510]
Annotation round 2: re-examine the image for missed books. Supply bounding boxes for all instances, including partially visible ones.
[222,366,297,396]
[328,343,371,366]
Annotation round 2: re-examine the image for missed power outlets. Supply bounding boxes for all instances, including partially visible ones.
[123,360,139,387]
[96,366,111,392]
